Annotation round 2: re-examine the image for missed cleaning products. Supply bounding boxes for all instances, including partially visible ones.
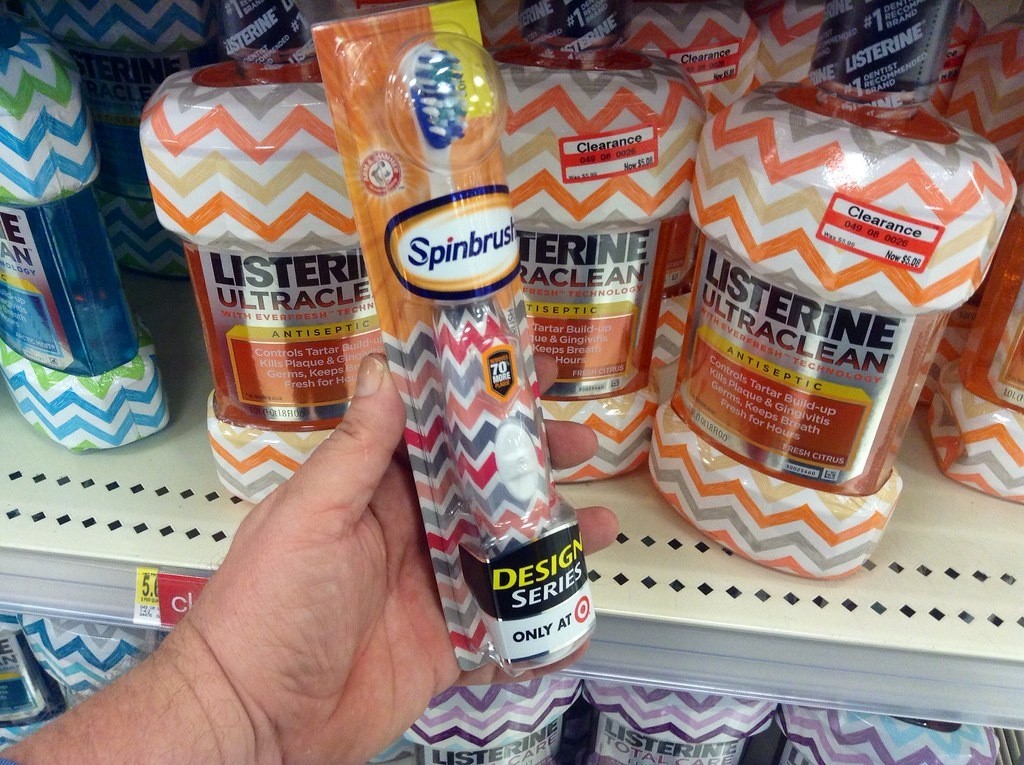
[0,1,1024,764]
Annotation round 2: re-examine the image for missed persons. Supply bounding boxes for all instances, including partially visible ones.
[0,352,620,765]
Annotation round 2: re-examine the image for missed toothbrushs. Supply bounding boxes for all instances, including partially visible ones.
[398,39,597,668]
